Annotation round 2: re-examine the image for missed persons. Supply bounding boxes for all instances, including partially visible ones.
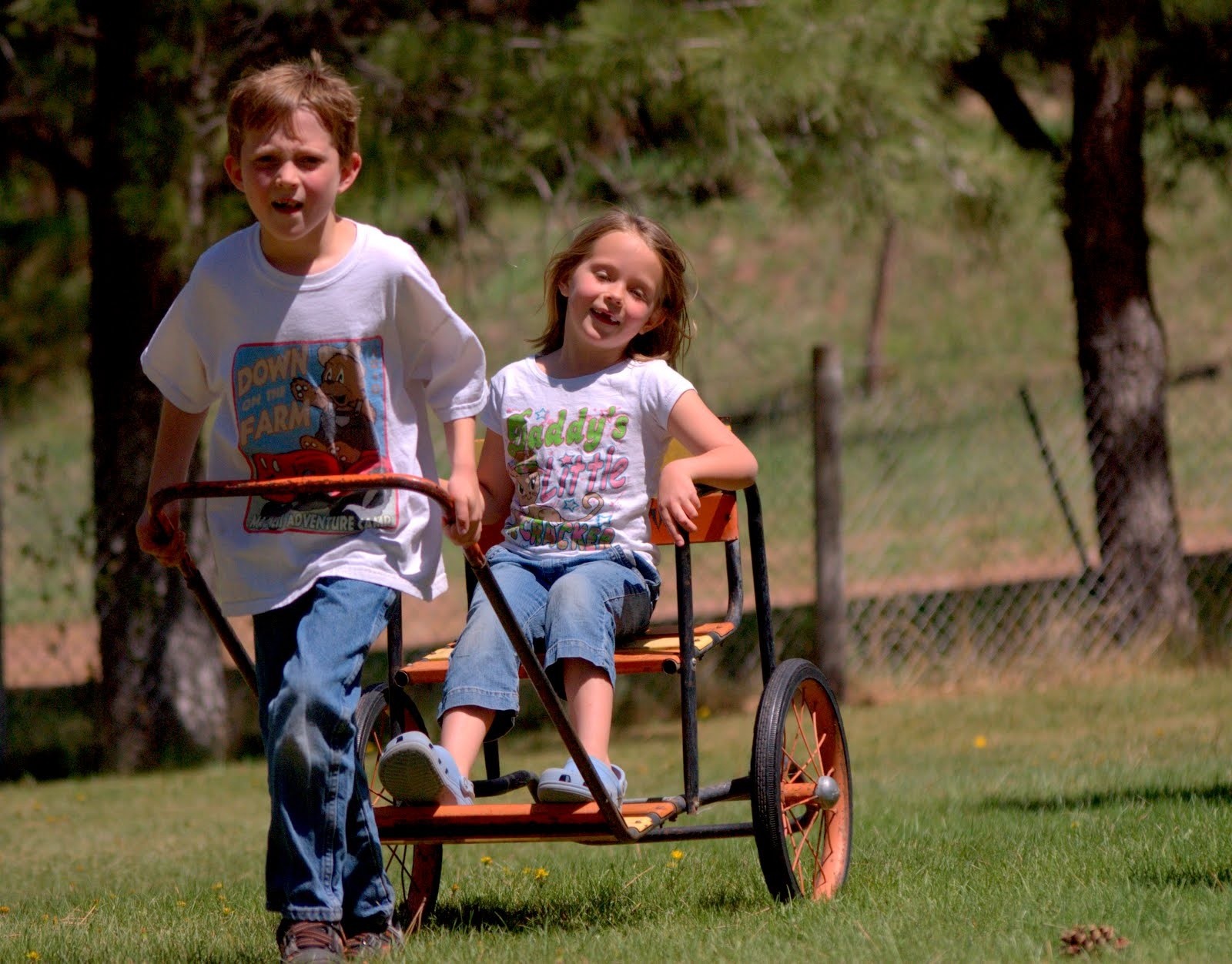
[378,212,759,812]
[138,54,490,964]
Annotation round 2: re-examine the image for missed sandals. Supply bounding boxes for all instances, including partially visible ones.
[538,756,627,814]
[377,731,475,807]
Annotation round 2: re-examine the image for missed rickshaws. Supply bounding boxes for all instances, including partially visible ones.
[151,415,857,937]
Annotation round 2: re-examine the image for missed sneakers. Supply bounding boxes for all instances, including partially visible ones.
[345,918,403,963]
[276,917,346,964]
